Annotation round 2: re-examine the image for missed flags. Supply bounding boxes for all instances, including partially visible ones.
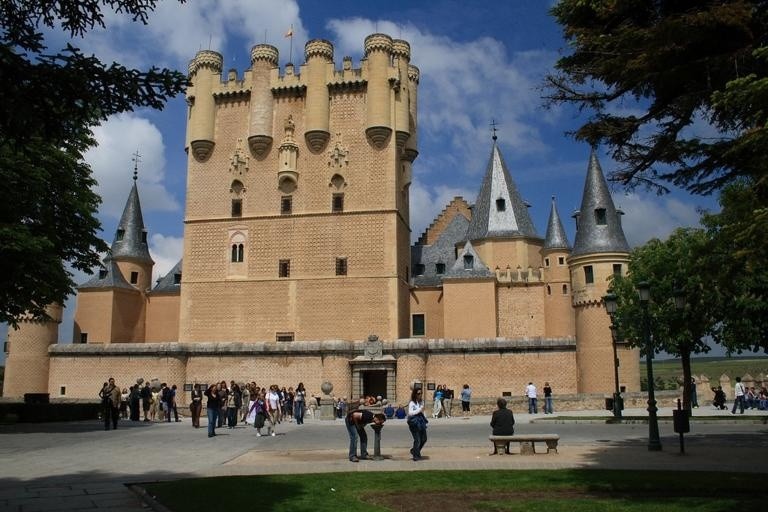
[284,31,292,37]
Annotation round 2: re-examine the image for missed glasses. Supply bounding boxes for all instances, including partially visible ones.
[416,392,423,395]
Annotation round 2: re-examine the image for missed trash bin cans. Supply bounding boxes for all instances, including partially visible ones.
[673,410,689,432]
[619,399,624,410]
[606,399,613,410]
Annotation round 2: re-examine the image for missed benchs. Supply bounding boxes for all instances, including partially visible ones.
[488,432,560,455]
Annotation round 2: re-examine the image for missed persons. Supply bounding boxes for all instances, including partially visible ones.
[526,382,537,414]
[407,387,428,461]
[691,377,700,409]
[189,380,306,438]
[307,394,318,420]
[460,384,471,419]
[542,382,553,414]
[711,377,768,415]
[432,384,452,419]
[490,398,515,454]
[99,377,181,430]
[332,394,406,462]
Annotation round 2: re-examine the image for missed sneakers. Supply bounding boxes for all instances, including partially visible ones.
[361,454,373,459]
[256,427,276,437]
[350,455,359,461]
